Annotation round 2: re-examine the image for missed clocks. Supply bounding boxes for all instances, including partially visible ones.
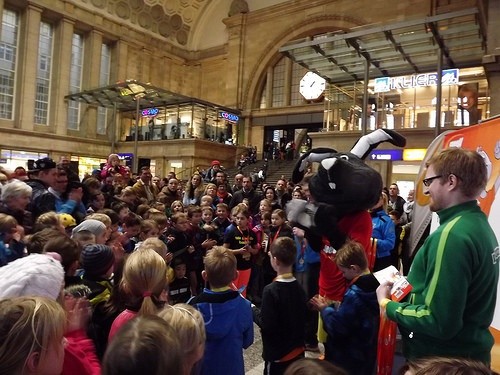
[299,72,327,101]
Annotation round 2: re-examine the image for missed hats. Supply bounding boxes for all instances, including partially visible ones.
[211,160,220,168]
[57,213,76,228]
[70,219,106,239]
[0,253,64,301]
[79,243,115,276]
[25,159,56,173]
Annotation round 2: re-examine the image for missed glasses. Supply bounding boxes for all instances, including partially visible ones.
[422,173,458,187]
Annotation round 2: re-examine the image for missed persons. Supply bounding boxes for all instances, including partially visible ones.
[0,138,500,375]
